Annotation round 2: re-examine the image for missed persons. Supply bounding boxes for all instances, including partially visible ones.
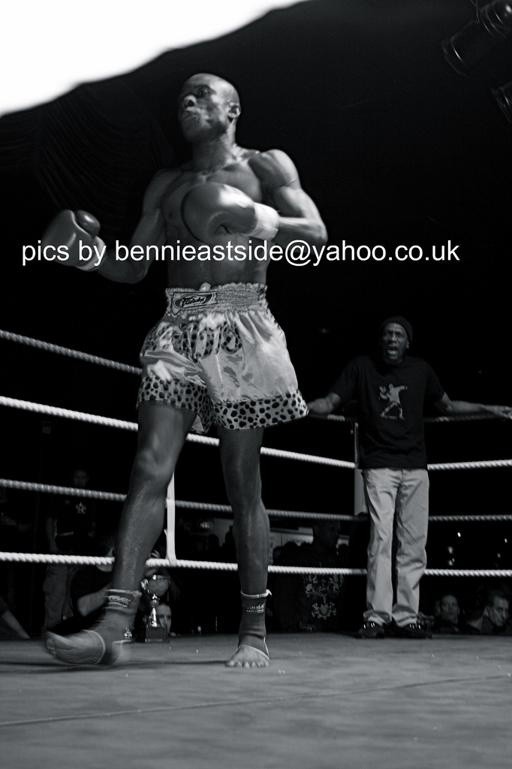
[0,467,512,640]
[307,317,512,639]
[40,74,329,669]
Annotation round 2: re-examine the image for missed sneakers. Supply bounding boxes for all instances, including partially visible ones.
[358,622,384,638]
[392,623,425,639]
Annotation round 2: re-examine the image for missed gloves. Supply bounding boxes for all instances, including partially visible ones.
[40,209,105,272]
[182,182,279,246]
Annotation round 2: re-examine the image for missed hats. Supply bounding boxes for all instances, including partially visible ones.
[380,317,412,341]
